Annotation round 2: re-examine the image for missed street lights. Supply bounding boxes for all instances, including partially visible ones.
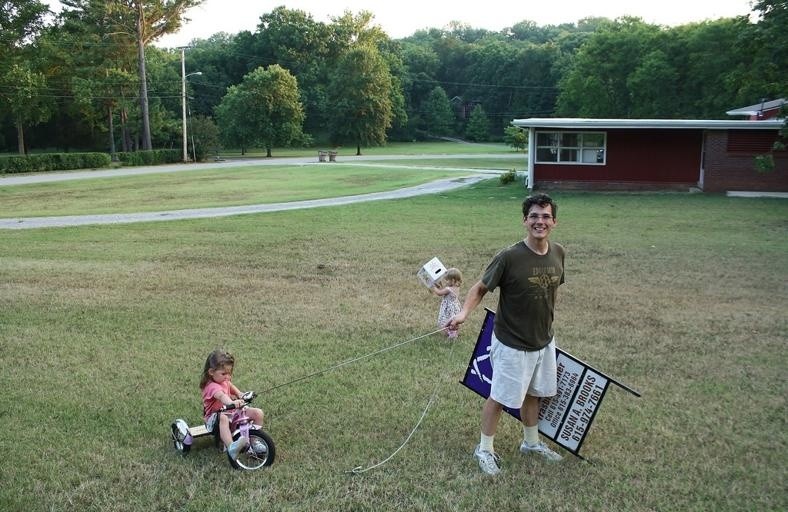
[181,71,203,163]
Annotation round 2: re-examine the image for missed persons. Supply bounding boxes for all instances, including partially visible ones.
[429,268,464,340]
[199,349,268,460]
[441,192,570,475]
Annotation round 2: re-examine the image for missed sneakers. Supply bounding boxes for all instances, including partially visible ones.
[248,440,267,453]
[520,440,563,462]
[474,442,502,475]
[447,331,458,339]
[227,435,247,460]
